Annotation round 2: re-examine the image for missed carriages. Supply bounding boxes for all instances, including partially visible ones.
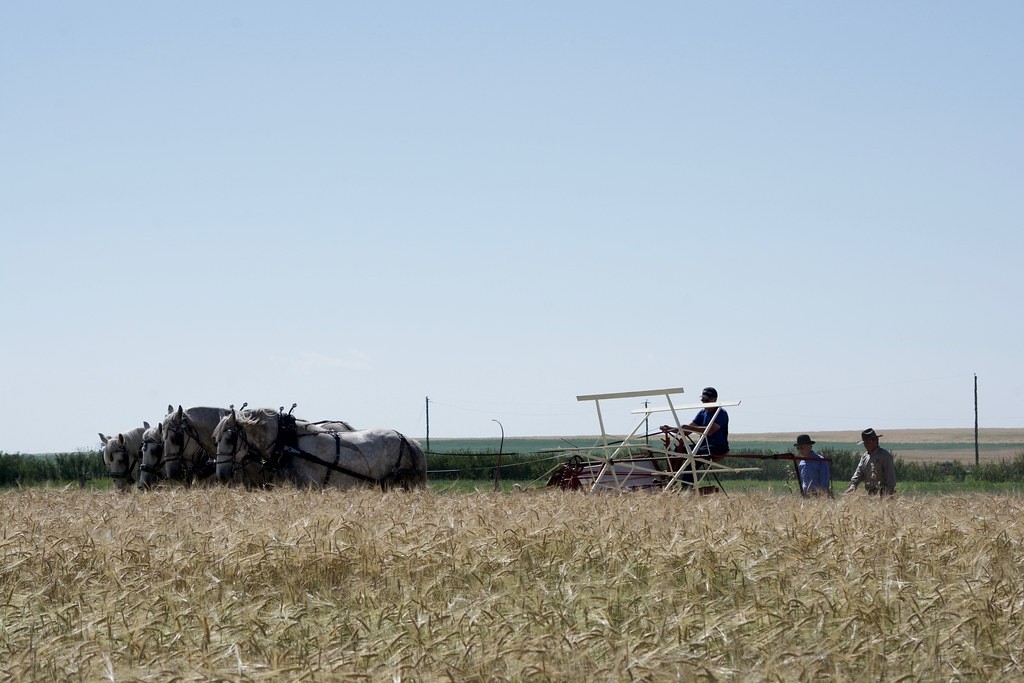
[97,386,765,495]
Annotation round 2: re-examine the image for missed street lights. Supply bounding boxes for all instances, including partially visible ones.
[425,396,431,452]
[973,372,980,467]
[642,399,650,444]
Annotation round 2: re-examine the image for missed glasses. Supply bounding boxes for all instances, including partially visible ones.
[797,445,809,450]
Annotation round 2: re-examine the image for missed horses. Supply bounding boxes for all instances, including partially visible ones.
[98,404,426,493]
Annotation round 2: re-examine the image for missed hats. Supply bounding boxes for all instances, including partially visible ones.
[856,428,883,445]
[699,387,718,400]
[793,435,816,447]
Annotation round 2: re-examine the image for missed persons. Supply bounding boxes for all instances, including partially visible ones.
[663,387,731,492]
[793,435,831,496]
[844,428,896,495]
